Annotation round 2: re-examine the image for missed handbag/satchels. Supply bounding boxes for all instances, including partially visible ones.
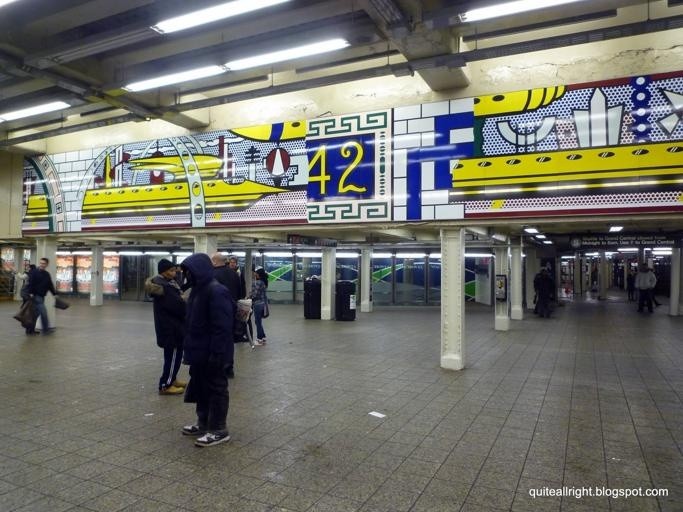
[263,303,270,317]
[14,303,37,325]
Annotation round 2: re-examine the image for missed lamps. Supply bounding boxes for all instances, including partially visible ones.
[0,0,579,127]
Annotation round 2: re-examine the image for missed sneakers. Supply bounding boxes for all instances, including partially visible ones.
[252,335,267,346]
[193,431,230,448]
[182,424,205,434]
[23,327,56,337]
[170,379,188,387]
[159,386,185,395]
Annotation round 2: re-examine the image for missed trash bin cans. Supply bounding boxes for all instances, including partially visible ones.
[303,278,321,319]
[335,280,356,321]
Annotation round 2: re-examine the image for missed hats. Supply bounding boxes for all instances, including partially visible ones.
[157,259,176,274]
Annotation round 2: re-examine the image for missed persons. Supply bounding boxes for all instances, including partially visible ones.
[174,256,270,346]
[535,268,554,318]
[21,257,59,336]
[146,256,187,396]
[534,269,546,314]
[13,264,36,309]
[179,253,234,446]
[613,262,664,313]
